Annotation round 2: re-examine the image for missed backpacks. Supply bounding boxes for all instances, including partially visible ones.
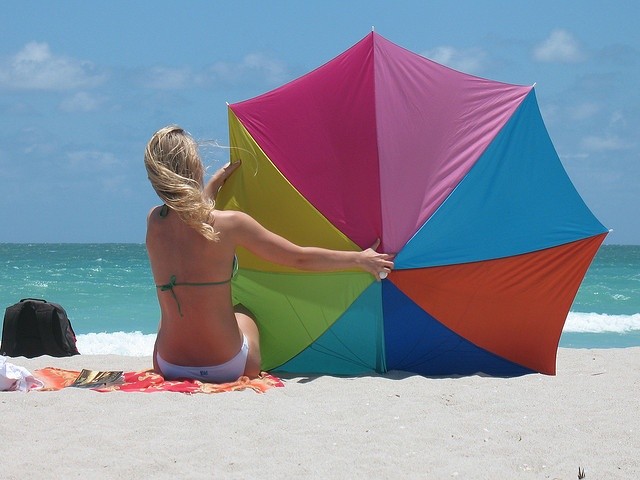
[0,298,81,359]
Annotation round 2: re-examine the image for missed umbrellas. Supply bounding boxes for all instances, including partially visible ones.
[214,26,614,374]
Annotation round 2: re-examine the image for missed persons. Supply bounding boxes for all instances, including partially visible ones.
[146,125,396,384]
